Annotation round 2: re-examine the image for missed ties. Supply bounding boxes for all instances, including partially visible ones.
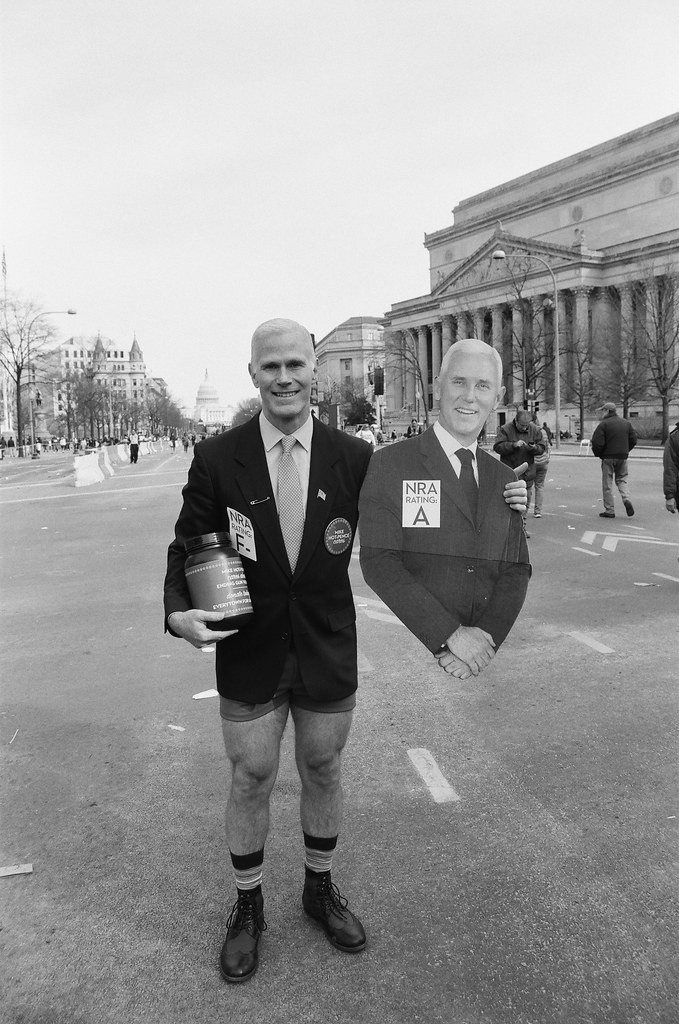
[454,448,479,530]
[278,435,305,575]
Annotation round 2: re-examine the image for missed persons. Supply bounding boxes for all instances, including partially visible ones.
[591,402,637,519]
[662,422,679,513]
[391,429,397,441]
[0,435,119,457]
[407,418,422,439]
[357,339,532,680]
[168,432,196,454]
[127,429,139,464]
[163,317,528,983]
[352,425,383,447]
[493,409,552,519]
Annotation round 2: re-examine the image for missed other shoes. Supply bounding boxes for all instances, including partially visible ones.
[624,500,635,517]
[599,512,616,518]
[533,513,542,518]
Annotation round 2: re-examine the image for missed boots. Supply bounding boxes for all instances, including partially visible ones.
[302,863,367,953]
[218,884,268,982]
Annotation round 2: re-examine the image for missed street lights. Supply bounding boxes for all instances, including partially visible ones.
[27,309,77,455]
[492,251,562,449]
[376,325,420,431]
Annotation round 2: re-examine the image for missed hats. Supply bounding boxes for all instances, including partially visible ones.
[596,402,616,412]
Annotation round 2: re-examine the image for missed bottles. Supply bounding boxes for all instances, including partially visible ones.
[184,532,254,631]
